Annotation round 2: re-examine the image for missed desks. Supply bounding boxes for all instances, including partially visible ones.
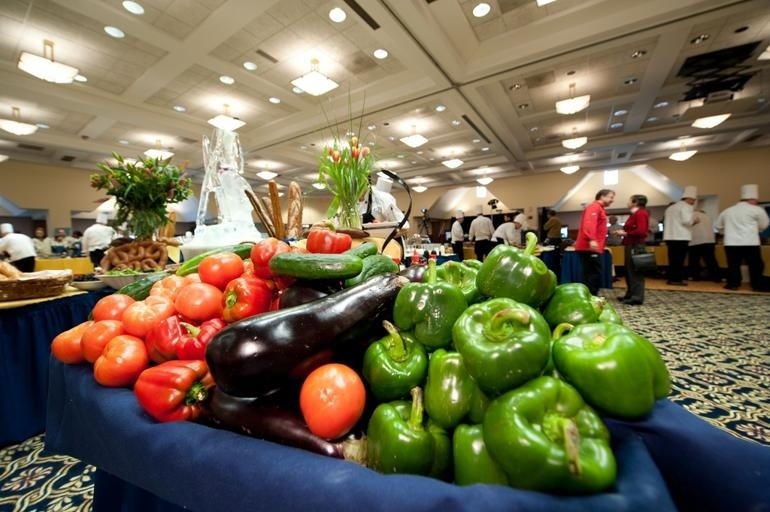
[46,363,770,511]
[402,254,459,269]
[0,283,104,456]
[541,251,614,292]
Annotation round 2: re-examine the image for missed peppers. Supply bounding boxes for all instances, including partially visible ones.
[134,229,353,422]
[364,232,672,494]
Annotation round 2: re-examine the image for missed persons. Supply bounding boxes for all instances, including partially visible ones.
[450,209,468,260]
[468,204,495,262]
[713,183,769,292]
[613,194,649,305]
[543,210,562,244]
[688,210,724,281]
[661,185,701,287]
[575,189,615,297]
[606,215,623,245]
[0,213,115,273]
[333,171,411,229]
[645,210,659,242]
[503,213,512,222]
[489,213,526,253]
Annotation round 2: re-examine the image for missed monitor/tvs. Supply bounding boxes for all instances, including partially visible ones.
[559,225,569,238]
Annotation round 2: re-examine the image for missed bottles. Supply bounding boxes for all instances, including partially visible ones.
[411,245,438,266]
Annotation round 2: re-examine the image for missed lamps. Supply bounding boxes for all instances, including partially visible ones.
[691,113,733,130]
[16,39,79,86]
[669,142,698,162]
[561,128,588,150]
[207,103,246,132]
[559,161,580,175]
[290,58,339,97]
[0,107,37,137]
[398,133,495,193]
[144,139,326,199]
[555,83,591,115]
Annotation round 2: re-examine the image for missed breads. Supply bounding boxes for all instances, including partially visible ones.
[99,242,168,275]
[0,260,24,279]
[0,274,7,281]
[243,180,303,241]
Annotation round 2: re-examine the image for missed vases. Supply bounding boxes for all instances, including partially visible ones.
[338,209,362,230]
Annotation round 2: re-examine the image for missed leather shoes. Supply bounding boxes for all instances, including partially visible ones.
[617,295,628,301]
[623,297,642,305]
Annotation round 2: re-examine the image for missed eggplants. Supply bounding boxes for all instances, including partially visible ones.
[204,260,429,457]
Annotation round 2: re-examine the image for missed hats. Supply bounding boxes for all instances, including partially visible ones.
[0,223,13,234]
[455,210,464,219]
[376,177,393,193]
[514,213,526,225]
[740,183,758,201]
[681,185,697,200]
[476,205,483,215]
[96,212,109,225]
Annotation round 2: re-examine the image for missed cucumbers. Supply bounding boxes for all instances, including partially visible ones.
[114,241,402,300]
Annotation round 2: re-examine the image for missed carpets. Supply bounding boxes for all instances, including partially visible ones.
[1,285,770,512]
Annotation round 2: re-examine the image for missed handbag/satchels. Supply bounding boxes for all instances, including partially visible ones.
[631,252,656,272]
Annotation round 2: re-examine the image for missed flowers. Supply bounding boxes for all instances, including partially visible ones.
[316,80,376,212]
[81,149,193,241]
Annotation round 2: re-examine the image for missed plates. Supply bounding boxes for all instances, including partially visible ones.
[70,281,106,292]
[93,275,150,290]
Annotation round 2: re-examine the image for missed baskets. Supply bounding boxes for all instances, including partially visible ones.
[0,273,69,302]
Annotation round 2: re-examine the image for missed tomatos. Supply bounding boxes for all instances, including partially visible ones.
[52,228,251,389]
